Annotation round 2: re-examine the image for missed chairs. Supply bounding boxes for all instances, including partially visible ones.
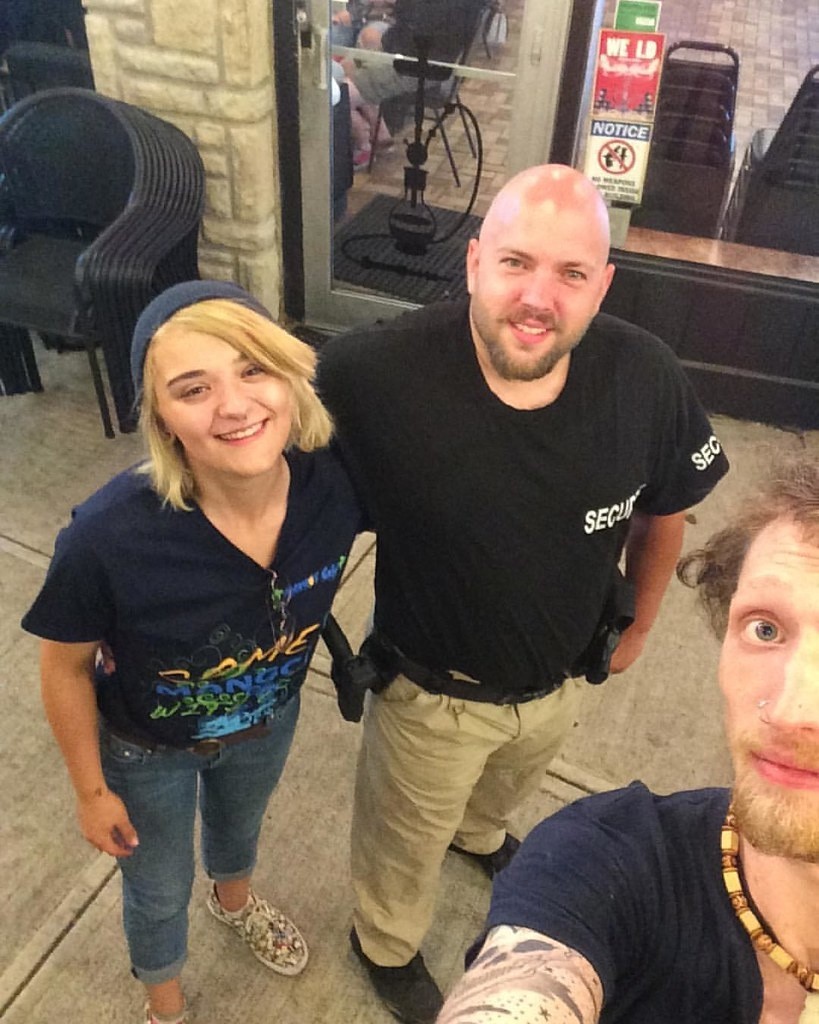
[1,87,204,436]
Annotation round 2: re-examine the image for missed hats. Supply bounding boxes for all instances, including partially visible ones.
[130,279,275,409]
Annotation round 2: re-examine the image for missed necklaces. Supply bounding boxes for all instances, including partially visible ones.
[718,792,819,1024]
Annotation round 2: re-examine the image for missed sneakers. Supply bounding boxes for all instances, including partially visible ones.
[448,833,522,881]
[205,877,308,976]
[144,992,187,1024]
[350,924,444,1024]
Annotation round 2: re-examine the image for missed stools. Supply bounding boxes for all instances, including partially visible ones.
[632,41,819,256]
[367,7,492,186]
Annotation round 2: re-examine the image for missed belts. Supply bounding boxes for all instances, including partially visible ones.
[98,697,299,755]
[370,627,590,706]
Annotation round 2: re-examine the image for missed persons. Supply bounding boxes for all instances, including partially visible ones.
[436,452,819,1024]
[306,164,729,1023]
[21,281,353,1024]
[318,0,483,170]
